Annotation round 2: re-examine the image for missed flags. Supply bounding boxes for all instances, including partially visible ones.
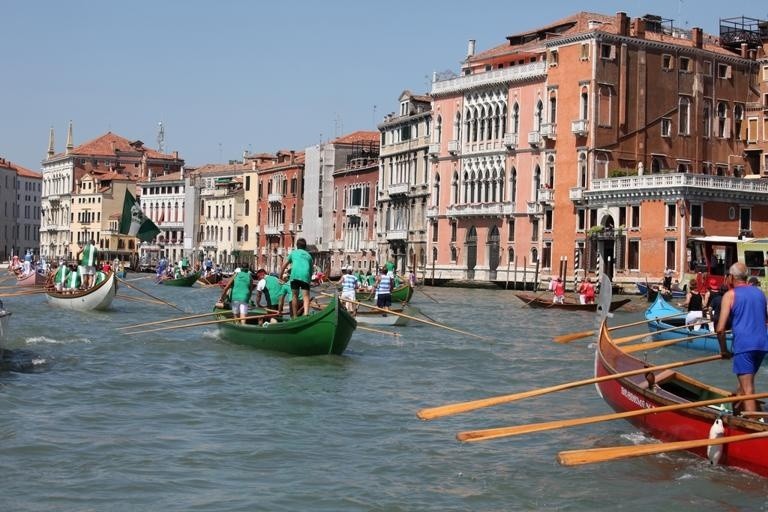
[118,188,162,243]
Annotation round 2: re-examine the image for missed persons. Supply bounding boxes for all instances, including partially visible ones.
[282,263,324,287]
[374,267,393,317]
[334,269,358,313]
[653,251,767,332]
[408,270,415,287]
[216,286,253,325]
[716,263,768,419]
[10,240,126,295]
[279,238,314,319]
[279,270,304,318]
[221,262,254,325]
[154,256,224,280]
[256,268,283,324]
[347,256,401,292]
[546,277,595,305]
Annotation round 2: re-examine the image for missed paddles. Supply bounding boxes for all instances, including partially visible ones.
[414,354,768,422]
[113,275,177,307]
[553,312,734,354]
[455,393,768,442]
[555,430,768,468]
[0,283,85,297]
